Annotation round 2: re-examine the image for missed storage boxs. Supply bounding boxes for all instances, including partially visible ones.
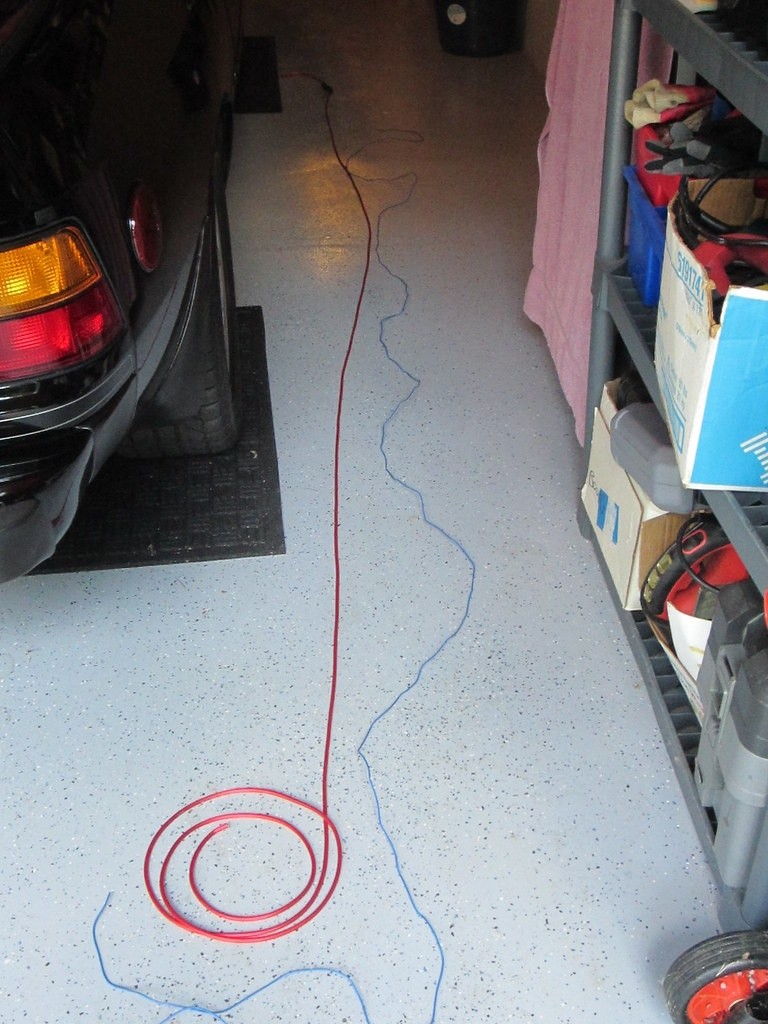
[622,125,767,492]
[581,376,714,726]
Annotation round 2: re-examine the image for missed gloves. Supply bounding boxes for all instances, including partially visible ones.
[620,78,715,129]
[641,115,766,179]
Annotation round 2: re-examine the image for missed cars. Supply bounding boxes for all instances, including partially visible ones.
[0,0,240,585]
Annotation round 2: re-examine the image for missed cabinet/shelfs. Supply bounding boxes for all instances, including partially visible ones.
[577,0,767,935]
